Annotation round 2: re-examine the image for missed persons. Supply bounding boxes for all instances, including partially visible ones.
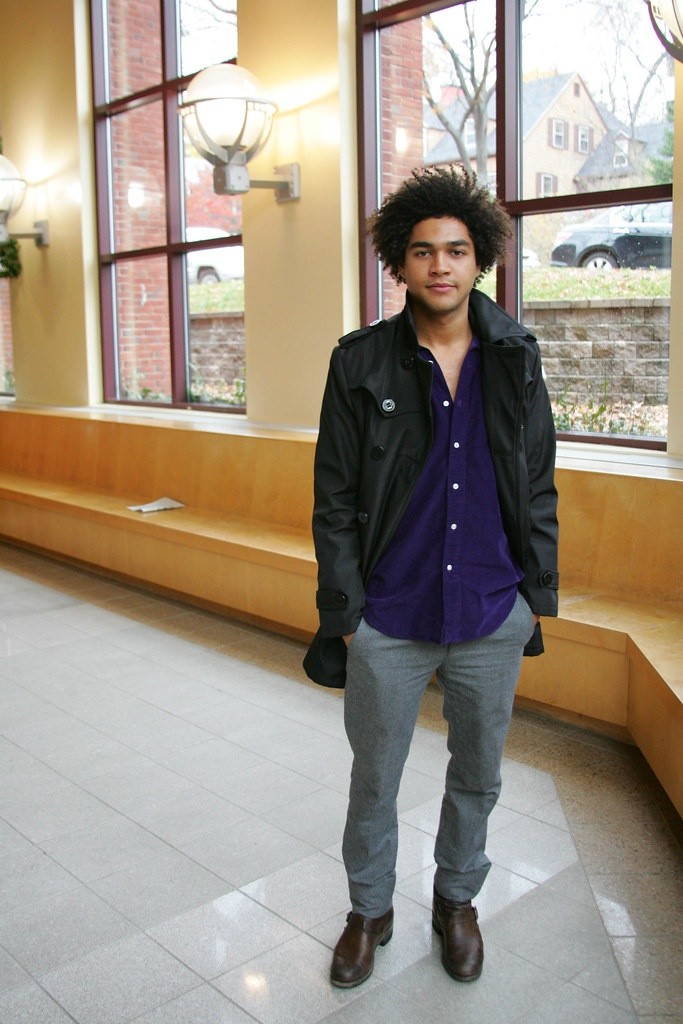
[312,176,560,988]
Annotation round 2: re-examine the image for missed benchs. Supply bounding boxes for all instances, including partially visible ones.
[0,406,683,842]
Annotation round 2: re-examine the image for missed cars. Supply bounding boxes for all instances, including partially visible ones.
[551,197,673,271]
[184,228,245,284]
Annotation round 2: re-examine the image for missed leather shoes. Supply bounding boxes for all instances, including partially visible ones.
[432,884,484,981]
[331,905,394,989]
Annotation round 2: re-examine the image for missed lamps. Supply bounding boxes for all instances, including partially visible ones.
[182,63,301,204]
[0,154,50,247]
